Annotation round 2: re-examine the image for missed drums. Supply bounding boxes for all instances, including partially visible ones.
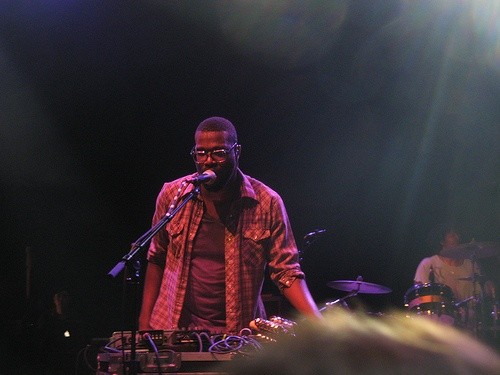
[403,282,459,327]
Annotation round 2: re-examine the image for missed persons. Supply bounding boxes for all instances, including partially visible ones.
[138,118,324,335]
[414,222,500,334]
[222,306,500,375]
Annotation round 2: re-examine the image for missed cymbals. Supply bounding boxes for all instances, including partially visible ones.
[455,273,500,283]
[437,240,500,260]
[327,280,393,294]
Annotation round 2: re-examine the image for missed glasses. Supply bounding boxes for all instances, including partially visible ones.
[190,142,237,164]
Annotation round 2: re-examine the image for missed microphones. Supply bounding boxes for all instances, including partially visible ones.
[185,169,217,185]
[304,228,326,239]
